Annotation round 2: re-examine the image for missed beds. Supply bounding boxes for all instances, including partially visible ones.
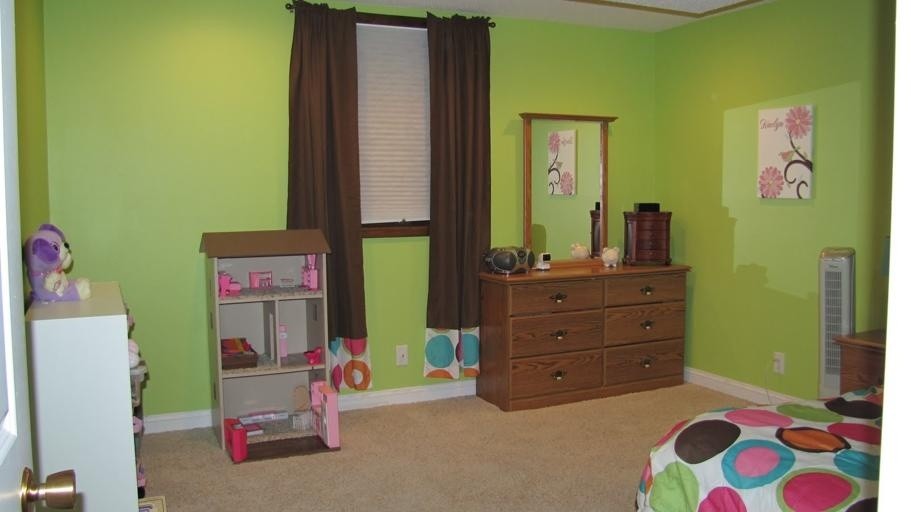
[637,385,886,511]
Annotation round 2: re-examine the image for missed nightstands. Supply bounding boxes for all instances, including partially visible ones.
[832,327,885,396]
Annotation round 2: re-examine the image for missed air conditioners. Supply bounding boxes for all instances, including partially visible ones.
[817,246,856,399]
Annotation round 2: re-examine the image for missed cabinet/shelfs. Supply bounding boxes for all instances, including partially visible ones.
[199,228,332,451]
[25,279,140,510]
[476,263,692,413]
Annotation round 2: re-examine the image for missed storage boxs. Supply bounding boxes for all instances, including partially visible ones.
[622,210,672,266]
[589,210,599,257]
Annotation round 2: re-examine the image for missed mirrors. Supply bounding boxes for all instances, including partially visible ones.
[518,112,618,269]
